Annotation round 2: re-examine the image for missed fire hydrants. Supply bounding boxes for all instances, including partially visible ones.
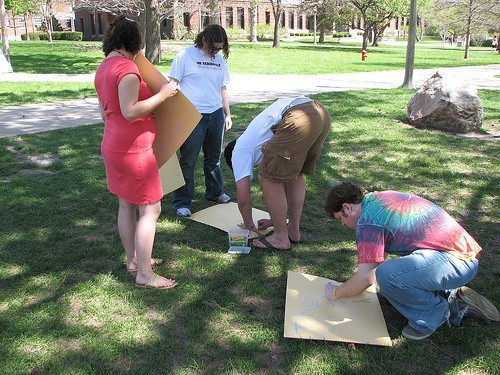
[360,49,368,62]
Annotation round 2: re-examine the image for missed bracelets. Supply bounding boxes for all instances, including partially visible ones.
[246,224,255,230]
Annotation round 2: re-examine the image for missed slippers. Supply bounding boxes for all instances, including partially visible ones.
[135,272,178,289]
[249,236,281,250]
[289,236,299,244]
[127,258,163,271]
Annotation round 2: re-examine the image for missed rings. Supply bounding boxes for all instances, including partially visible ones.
[173,90,176,92]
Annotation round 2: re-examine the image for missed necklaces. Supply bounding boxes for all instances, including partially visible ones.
[113,50,127,59]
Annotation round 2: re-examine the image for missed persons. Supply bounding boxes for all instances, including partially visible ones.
[168,25,232,216]
[94,15,180,289]
[224,94,331,250]
[324,182,500,340]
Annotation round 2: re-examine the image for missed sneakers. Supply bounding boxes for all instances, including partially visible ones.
[209,193,231,203]
[172,206,191,217]
[454,286,500,321]
[402,310,450,340]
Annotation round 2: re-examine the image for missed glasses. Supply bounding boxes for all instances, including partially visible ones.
[214,47,223,51]
[139,42,144,49]
